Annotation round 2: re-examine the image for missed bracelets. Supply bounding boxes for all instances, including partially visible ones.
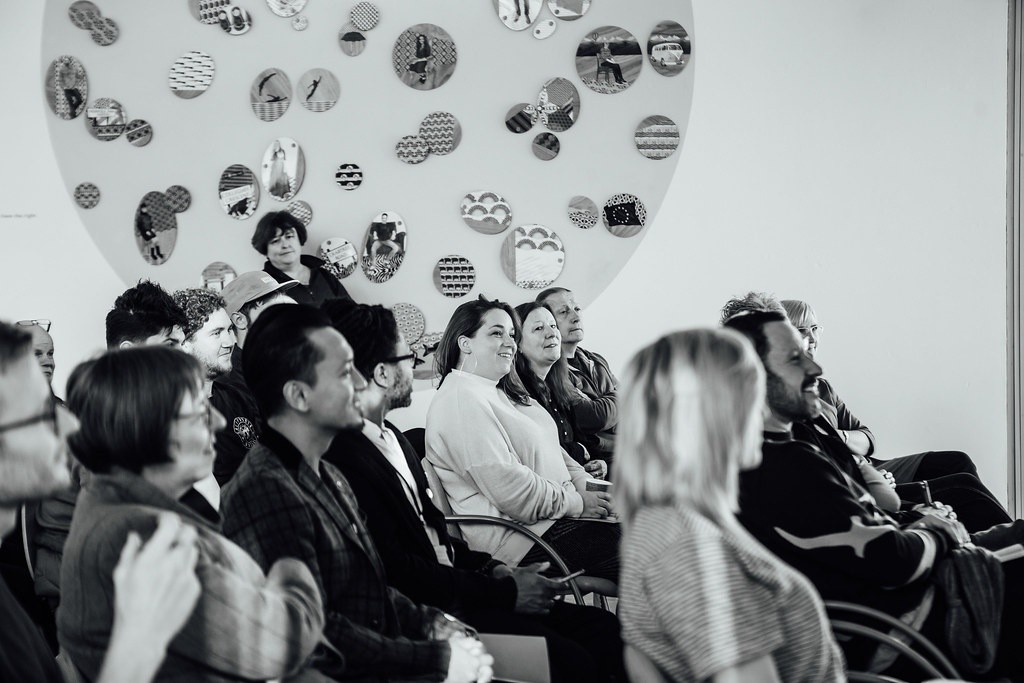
[843,430,849,444]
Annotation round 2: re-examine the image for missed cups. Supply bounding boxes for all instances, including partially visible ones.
[586,479,612,491]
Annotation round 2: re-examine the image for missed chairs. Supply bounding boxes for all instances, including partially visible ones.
[24,493,964,683]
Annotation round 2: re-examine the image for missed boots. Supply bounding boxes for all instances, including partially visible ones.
[156,246,163,258]
[151,248,157,260]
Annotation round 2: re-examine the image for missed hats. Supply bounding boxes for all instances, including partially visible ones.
[138,202,150,211]
[221,271,301,320]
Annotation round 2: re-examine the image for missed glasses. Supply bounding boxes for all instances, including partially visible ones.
[0,394,58,435]
[175,405,211,426]
[370,349,418,378]
[15,319,51,332]
[798,326,823,334]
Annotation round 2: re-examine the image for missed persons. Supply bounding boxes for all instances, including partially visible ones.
[0,278,1024,683]
[252,212,352,305]
[513,0,531,24]
[369,214,399,270]
[599,37,627,84]
[408,35,433,84]
[58,58,84,119]
[269,149,291,200]
[137,204,164,260]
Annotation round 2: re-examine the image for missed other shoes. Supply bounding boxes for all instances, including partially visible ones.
[615,78,628,85]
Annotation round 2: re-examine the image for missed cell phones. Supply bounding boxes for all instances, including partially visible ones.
[555,568,587,583]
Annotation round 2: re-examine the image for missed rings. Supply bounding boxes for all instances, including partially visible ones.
[595,472,598,477]
